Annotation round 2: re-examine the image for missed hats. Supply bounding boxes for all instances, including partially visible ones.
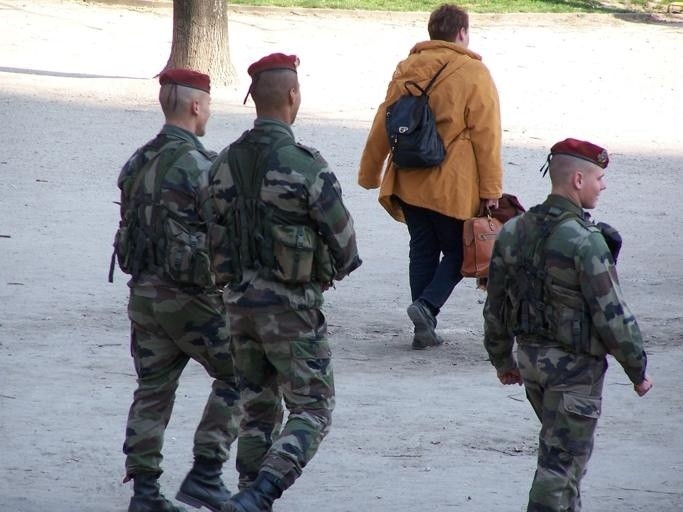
[159,68,210,95]
[248,53,299,76]
[552,139,609,170]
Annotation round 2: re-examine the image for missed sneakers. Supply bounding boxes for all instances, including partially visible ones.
[407,300,439,346]
[411,335,442,349]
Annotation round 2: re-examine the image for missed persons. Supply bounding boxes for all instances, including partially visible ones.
[358,4,502,349]
[208,53,362,512]
[115,70,245,512]
[482,139,654,512]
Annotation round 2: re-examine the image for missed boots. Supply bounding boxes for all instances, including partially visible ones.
[176,455,230,511]
[129,473,184,511]
[224,474,280,511]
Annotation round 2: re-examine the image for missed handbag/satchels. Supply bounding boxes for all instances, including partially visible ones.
[462,207,501,277]
[385,95,446,169]
[485,194,524,223]
